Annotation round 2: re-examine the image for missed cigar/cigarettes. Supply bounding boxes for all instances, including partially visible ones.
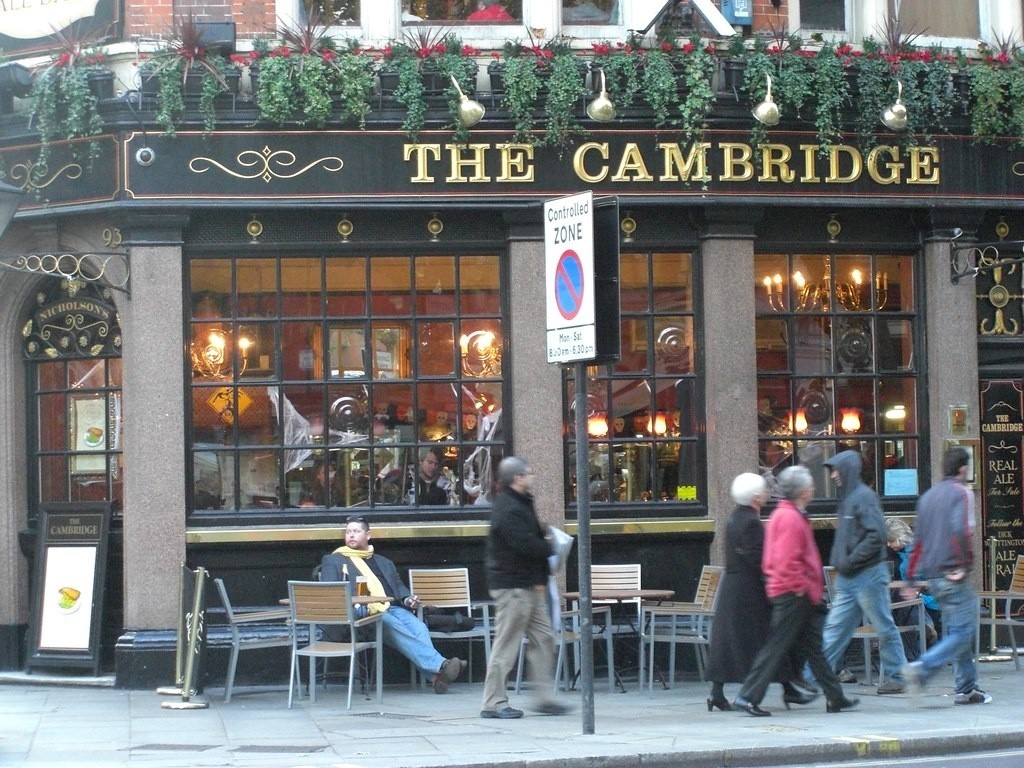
[411,597,419,603]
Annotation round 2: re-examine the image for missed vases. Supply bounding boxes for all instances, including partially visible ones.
[139,67,162,97]
[181,68,204,93]
[222,68,242,91]
[579,65,590,91]
[605,69,623,92]
[378,72,400,95]
[722,57,745,89]
[532,71,552,93]
[668,58,687,89]
[489,70,505,95]
[466,69,478,94]
[86,68,115,99]
[421,69,433,96]
[842,66,860,93]
[915,70,928,93]
[434,76,449,95]
[950,73,970,94]
[248,66,262,93]
[589,62,606,91]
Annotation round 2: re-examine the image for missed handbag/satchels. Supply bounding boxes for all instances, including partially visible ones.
[422,603,476,632]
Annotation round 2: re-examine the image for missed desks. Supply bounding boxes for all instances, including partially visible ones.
[561,587,680,695]
[273,595,394,702]
[823,577,930,688]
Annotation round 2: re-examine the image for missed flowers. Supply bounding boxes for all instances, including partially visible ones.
[20,29,1024,180]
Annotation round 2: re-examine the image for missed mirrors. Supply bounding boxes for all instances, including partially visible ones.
[312,321,412,379]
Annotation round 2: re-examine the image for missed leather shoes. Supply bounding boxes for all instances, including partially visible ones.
[733,696,772,717]
[827,697,860,713]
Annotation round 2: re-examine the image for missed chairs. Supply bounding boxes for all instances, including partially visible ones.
[513,601,618,699]
[972,554,1024,676]
[212,577,306,704]
[823,565,928,684]
[282,578,388,711]
[638,562,717,682]
[640,565,728,699]
[406,568,491,690]
[569,559,648,686]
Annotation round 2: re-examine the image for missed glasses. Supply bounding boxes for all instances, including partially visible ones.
[346,516,367,523]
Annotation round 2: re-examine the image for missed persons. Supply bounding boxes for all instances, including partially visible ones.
[478,456,583,719]
[880,513,941,660]
[730,464,862,715]
[383,445,452,506]
[299,458,349,509]
[836,661,857,683]
[902,447,993,705]
[799,449,911,694]
[703,471,819,712]
[319,516,468,694]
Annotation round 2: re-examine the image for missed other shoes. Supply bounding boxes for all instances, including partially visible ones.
[481,708,524,719]
[839,668,857,683]
[529,703,572,716]
[953,690,993,705]
[924,624,939,645]
[431,657,467,693]
[876,681,906,695]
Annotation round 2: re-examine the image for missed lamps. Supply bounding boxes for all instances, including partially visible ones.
[0,61,38,99]
[750,66,782,128]
[877,77,910,133]
[447,71,487,129]
[645,407,671,445]
[836,405,861,446]
[193,294,262,379]
[459,327,502,378]
[588,408,610,439]
[882,403,906,421]
[177,19,241,57]
[585,66,618,124]
[781,405,810,448]
[761,255,890,314]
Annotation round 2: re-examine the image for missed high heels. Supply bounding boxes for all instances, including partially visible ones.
[783,688,817,710]
[707,697,732,711]
